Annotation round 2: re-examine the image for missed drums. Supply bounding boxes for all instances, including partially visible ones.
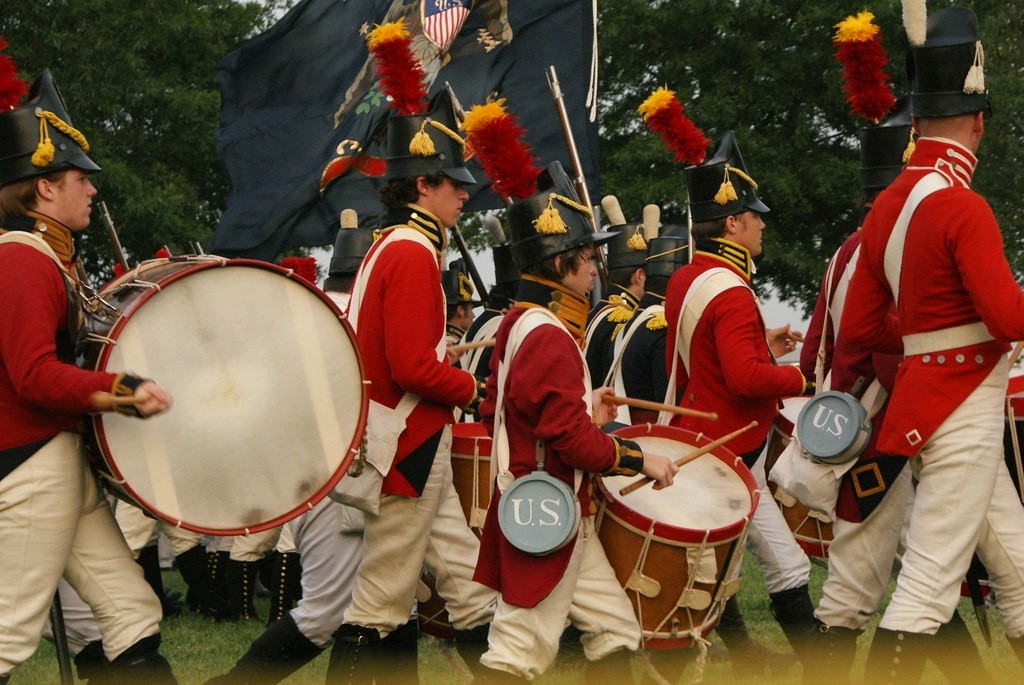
[71,253,369,537]
[1004,368,1024,507]
[449,423,493,540]
[591,423,759,652]
[765,397,834,558]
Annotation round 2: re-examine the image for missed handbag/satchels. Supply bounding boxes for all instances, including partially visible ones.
[329,398,406,516]
[767,441,860,522]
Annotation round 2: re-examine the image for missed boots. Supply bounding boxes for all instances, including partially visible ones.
[373,618,419,685]
[324,624,380,685]
[200,612,324,685]
[442,589,1024,685]
[106,632,178,685]
[133,546,301,630]
[73,639,113,685]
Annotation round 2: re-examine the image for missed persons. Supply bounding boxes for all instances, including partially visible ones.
[801,1,1024,685]
[199,18,822,685]
[0,35,180,684]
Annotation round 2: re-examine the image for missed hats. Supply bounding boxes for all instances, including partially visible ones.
[0,35,103,187]
[834,11,915,188]
[325,17,772,304]
[902,0,989,117]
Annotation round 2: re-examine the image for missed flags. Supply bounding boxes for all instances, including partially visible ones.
[212,0,604,264]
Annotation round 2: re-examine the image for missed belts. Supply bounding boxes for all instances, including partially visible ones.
[903,323,995,355]
